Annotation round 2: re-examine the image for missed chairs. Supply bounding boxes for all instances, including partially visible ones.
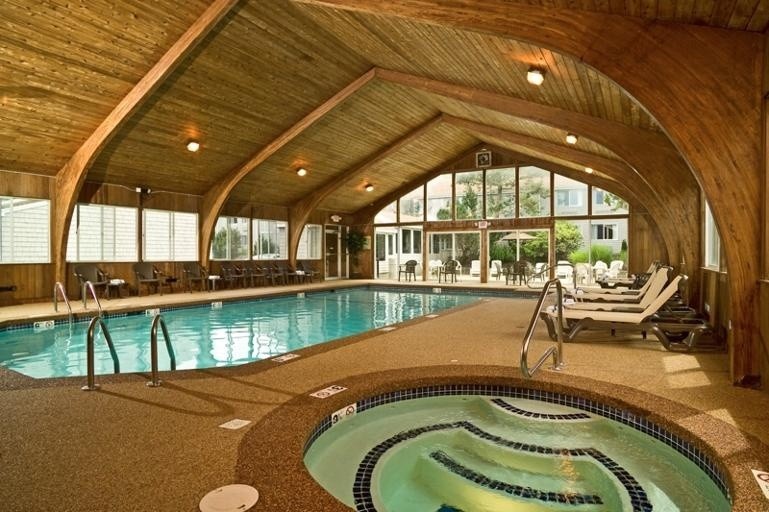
[73,259,322,302]
[540,259,708,353]
[421,259,624,287]
[398,260,417,282]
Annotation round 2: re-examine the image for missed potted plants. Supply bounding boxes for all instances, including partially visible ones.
[338,229,369,279]
